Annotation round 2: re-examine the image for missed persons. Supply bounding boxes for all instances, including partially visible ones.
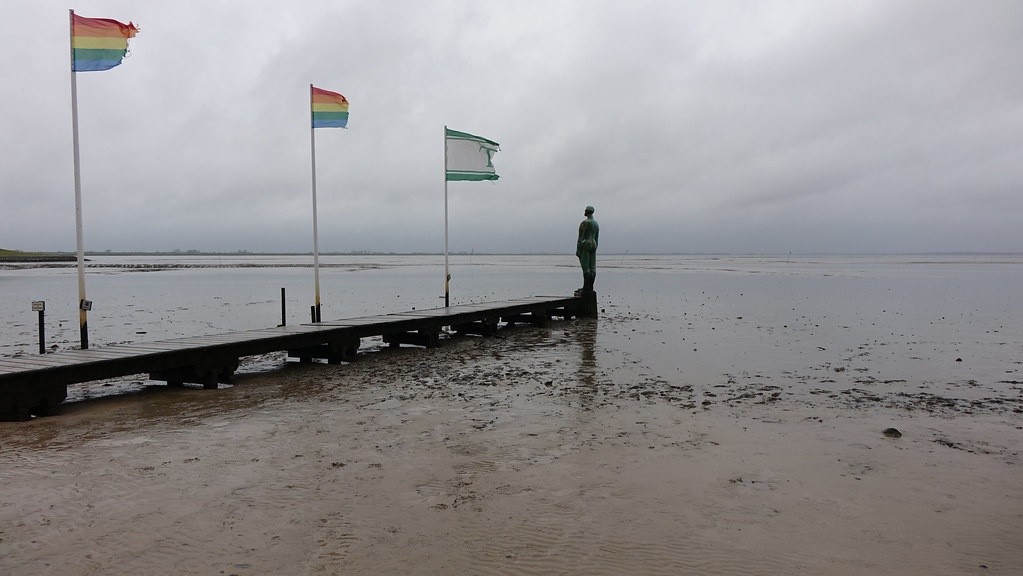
[576,206,599,291]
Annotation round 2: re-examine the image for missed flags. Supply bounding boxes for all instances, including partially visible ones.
[312,88,349,129]
[73,14,138,71]
[446,129,500,181]
